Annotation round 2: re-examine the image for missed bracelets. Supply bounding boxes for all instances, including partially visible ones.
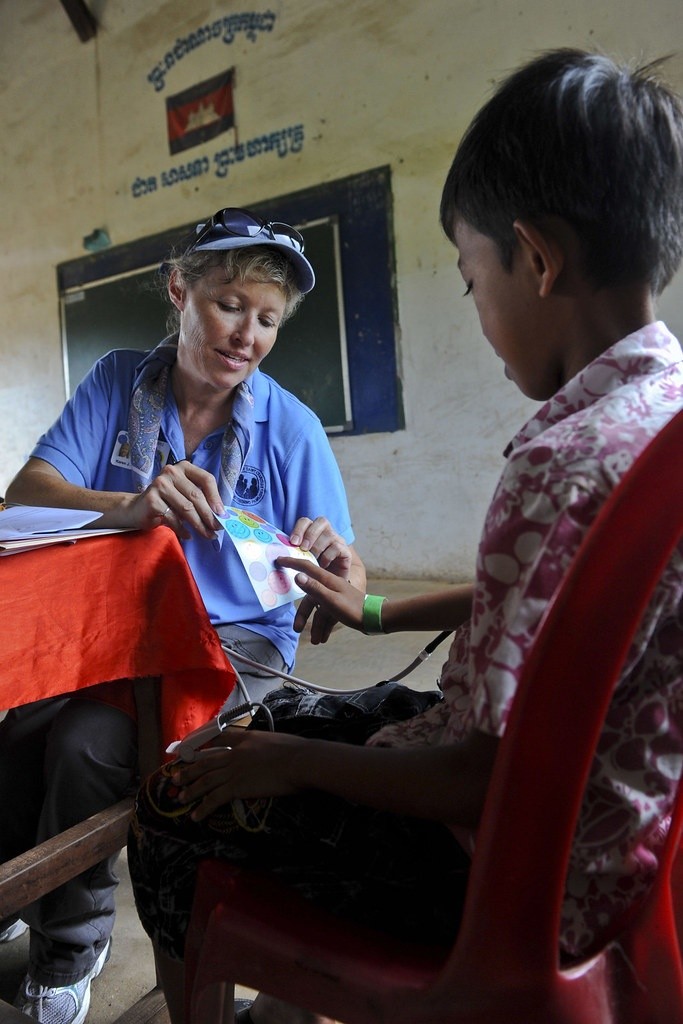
[362,595,388,638]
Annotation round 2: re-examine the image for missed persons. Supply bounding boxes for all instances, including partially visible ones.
[0,210,365,1024]
[127,47,683,1024]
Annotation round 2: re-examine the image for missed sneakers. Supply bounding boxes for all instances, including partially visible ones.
[11,935,112,1024]
[0,918,30,943]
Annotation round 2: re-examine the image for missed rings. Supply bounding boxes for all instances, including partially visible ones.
[161,506,172,516]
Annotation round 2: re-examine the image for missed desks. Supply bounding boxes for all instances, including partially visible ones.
[0,505,236,1024]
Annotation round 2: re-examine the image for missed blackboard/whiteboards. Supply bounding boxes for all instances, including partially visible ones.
[58,212,354,434]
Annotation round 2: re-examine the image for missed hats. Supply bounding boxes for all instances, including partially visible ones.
[158,222,315,294]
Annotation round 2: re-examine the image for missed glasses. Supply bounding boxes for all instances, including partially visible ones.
[184,207,305,254]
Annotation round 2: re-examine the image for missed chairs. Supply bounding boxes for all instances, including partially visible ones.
[180,409,683,1024]
